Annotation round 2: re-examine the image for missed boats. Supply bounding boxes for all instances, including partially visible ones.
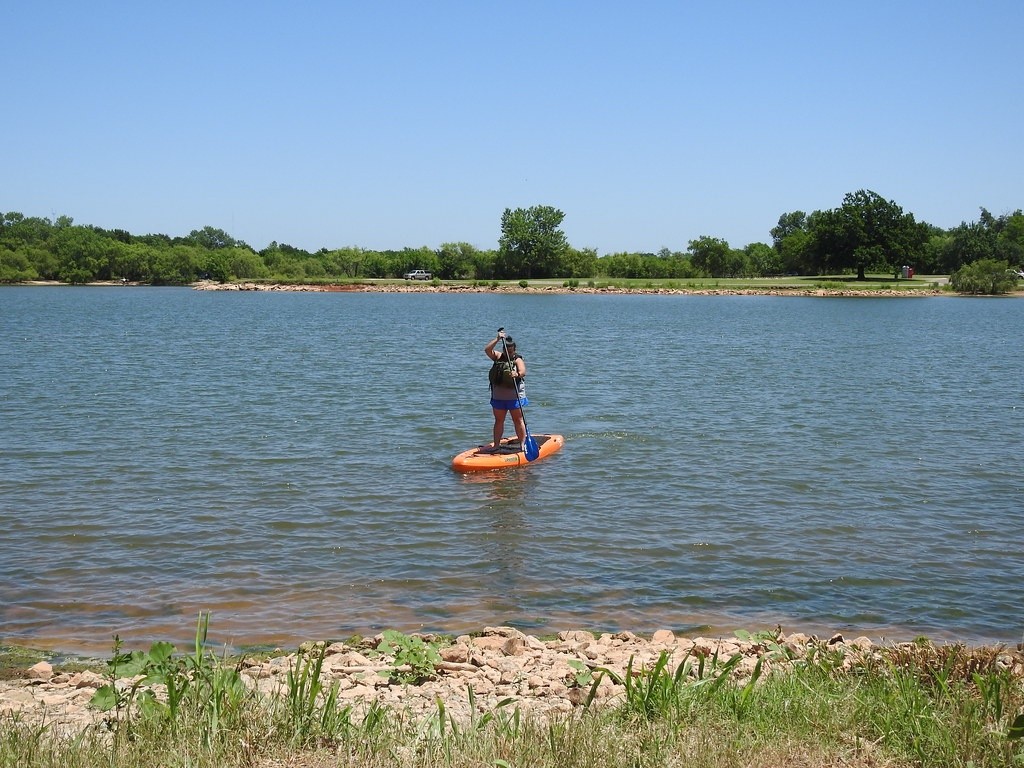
[451,433,564,473]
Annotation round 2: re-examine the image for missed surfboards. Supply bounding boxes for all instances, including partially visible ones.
[451,433,562,473]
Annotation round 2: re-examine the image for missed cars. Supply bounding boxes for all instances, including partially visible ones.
[1004,268,1024,279]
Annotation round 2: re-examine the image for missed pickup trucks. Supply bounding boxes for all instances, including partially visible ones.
[403,269,432,280]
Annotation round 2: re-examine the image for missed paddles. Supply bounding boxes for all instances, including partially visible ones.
[497,326,539,462]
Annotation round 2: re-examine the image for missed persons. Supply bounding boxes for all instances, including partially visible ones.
[485,331,529,451]
[121,277,127,287]
